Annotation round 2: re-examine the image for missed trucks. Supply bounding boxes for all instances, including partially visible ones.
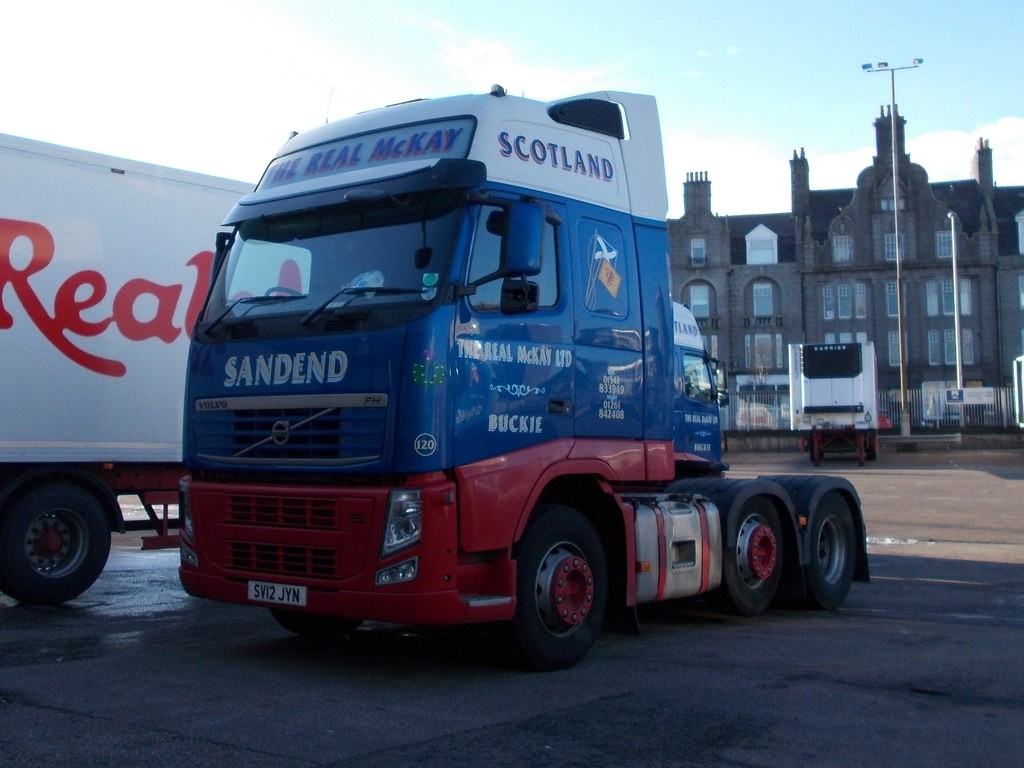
[1,130,733,610]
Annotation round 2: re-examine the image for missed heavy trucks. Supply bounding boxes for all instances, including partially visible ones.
[180,82,872,674]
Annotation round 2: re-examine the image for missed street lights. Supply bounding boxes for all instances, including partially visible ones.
[862,57,925,437]
